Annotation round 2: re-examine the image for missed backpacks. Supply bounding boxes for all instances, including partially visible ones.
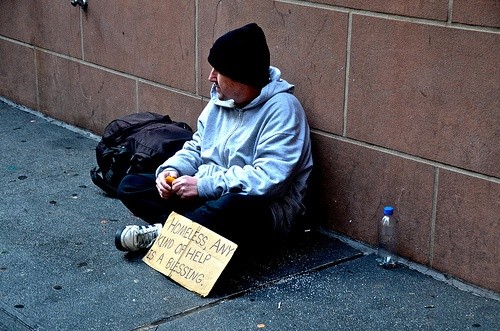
[90,111,193,197]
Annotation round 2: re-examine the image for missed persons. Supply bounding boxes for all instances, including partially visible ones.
[114,27,314,253]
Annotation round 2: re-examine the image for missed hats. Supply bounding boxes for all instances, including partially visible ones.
[208,22,271,87]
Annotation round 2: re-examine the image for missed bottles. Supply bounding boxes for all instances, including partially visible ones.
[377,207,398,266]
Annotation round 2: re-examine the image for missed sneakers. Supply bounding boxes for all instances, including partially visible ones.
[114,222,165,253]
